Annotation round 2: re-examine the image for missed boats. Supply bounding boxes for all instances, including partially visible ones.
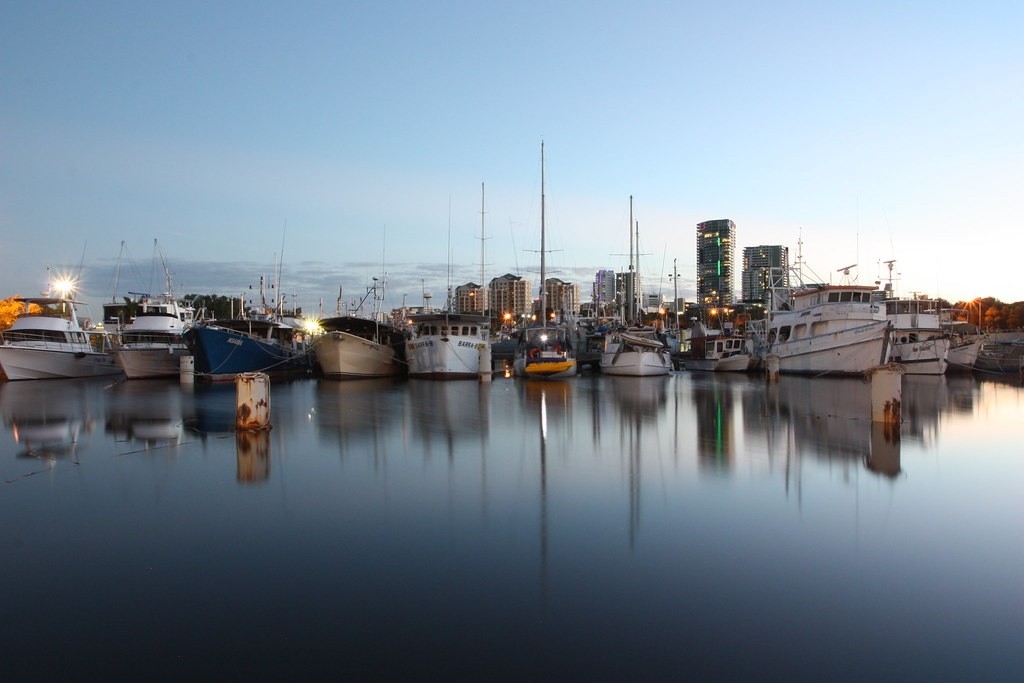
[0,383,109,484]
[900,308,979,372]
[0,298,123,379]
[180,318,318,382]
[313,315,404,381]
[673,334,750,372]
[405,313,491,378]
[967,326,1024,372]
[492,324,577,377]
[103,375,196,462]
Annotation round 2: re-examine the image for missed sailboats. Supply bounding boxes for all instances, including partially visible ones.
[749,226,895,378]
[882,259,954,376]
[112,237,192,378]
[601,193,673,376]
[101,241,152,344]
